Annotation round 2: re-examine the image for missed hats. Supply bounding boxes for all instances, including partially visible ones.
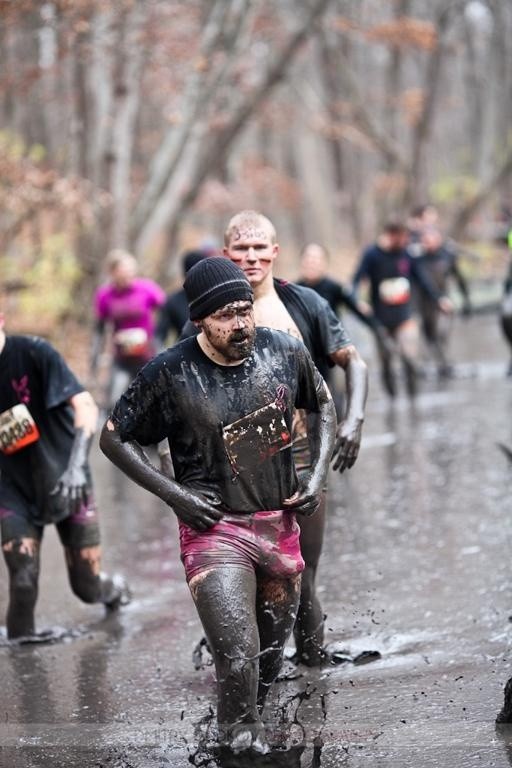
[183,256,254,321]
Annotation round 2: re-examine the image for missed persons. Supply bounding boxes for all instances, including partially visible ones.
[292,206,470,417]
[177,209,370,666]
[98,255,337,741]
[93,249,170,417]
[0,311,126,641]
[150,242,223,358]
